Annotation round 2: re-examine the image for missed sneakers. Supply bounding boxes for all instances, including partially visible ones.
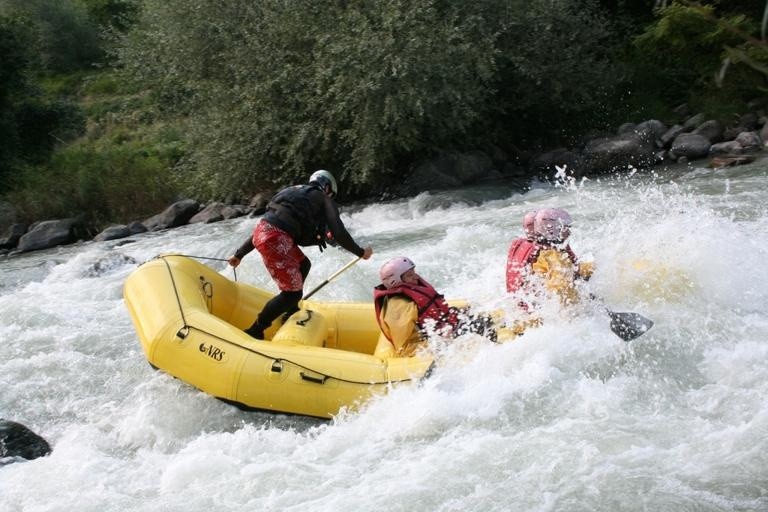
[282,310,301,323]
[244,327,264,341]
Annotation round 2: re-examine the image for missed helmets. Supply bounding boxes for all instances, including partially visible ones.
[378,257,417,290]
[533,208,573,241]
[309,170,338,195]
[523,208,539,241]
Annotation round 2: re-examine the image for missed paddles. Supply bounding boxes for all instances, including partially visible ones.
[590,293,654,341]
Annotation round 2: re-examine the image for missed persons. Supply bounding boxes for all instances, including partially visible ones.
[513,206,598,317]
[368,257,498,359]
[227,169,375,340]
[505,211,543,299]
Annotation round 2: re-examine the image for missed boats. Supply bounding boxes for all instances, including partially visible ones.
[124,254,698,420]
